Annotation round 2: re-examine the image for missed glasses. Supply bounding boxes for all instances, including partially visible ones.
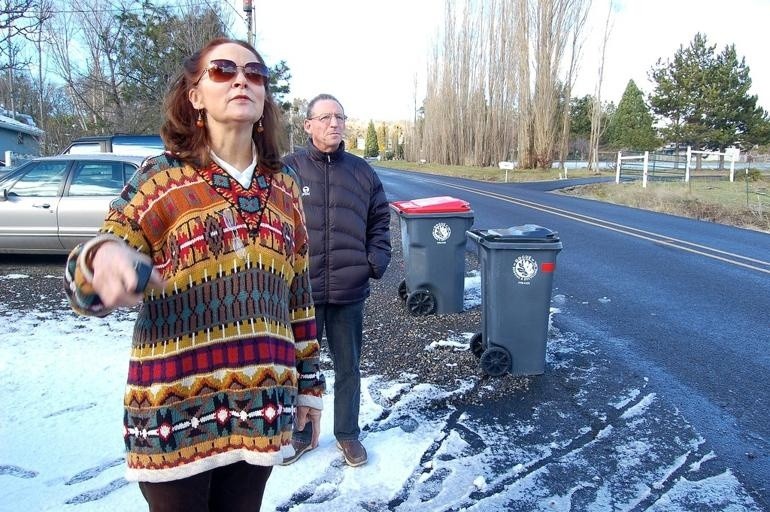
[308,114,347,124]
[192,60,270,87]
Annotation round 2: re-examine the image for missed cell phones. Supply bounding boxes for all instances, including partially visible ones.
[130,259,153,294]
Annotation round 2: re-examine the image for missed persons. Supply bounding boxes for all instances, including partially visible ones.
[279,95,391,467]
[64,39,327,512]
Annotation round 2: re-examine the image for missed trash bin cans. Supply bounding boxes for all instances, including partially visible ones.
[389,196,563,376]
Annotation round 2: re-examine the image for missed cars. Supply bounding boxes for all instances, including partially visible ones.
[0,134,165,255]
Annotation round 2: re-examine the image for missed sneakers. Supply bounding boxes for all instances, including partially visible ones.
[335,429,368,467]
[277,421,320,466]
